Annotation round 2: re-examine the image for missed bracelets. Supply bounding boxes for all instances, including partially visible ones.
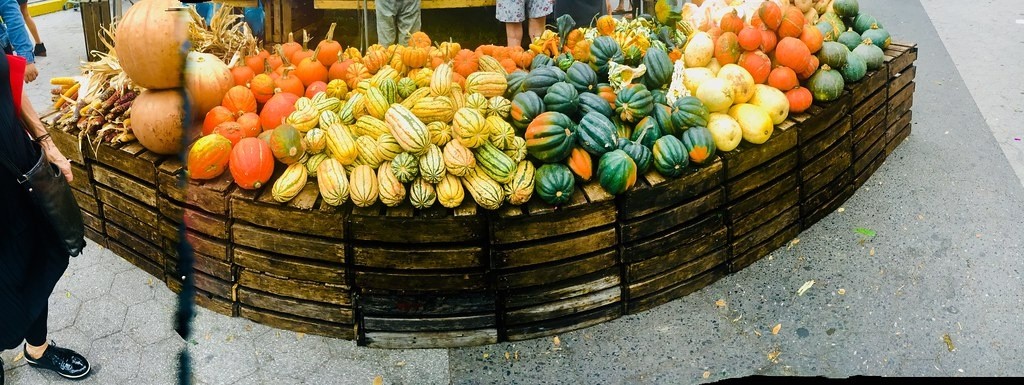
[35,133,51,150]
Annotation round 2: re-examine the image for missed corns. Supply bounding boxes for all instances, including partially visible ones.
[50,71,139,144]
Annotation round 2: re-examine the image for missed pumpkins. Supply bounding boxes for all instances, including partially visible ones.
[115,1,537,212]
[503,1,890,204]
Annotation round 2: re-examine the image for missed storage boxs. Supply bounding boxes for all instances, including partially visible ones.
[34,37,918,349]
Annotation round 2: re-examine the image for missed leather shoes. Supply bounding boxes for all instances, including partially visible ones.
[23,340,91,380]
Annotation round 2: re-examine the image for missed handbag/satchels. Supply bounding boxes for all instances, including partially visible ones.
[5,54,27,121]
[17,142,86,257]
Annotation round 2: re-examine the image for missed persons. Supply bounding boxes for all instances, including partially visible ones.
[17,0,47,56]
[376,0,421,49]
[0,0,38,83]
[0,51,91,385]
[612,0,632,19]
[496,0,554,46]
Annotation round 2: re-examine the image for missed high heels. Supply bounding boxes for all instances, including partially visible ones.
[34,43,46,56]
